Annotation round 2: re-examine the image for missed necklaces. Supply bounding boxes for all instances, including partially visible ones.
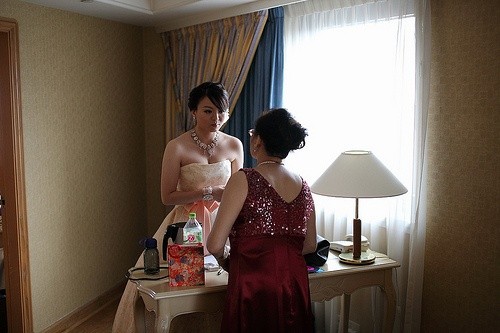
[257,161,276,167]
[190,127,219,150]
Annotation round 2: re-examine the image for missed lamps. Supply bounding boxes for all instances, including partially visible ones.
[310,150,408,267]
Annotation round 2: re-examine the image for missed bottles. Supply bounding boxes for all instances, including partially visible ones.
[143,238,160,273]
[182,213,202,244]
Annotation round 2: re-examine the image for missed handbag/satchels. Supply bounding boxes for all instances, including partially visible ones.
[167,244,205,287]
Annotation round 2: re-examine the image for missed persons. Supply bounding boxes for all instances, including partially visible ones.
[111,82,245,333]
[207,108,317,333]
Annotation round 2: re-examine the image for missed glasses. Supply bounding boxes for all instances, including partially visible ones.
[249,129,261,139]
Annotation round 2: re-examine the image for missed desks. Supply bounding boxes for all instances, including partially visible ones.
[127,249,402,333]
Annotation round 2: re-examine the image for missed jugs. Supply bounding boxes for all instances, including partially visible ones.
[162,222,202,260]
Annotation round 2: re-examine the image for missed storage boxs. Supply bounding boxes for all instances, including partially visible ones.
[167,244,206,287]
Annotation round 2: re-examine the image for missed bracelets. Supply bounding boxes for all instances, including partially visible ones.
[202,186,213,201]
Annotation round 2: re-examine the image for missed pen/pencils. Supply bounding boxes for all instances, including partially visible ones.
[218,269,224,275]
